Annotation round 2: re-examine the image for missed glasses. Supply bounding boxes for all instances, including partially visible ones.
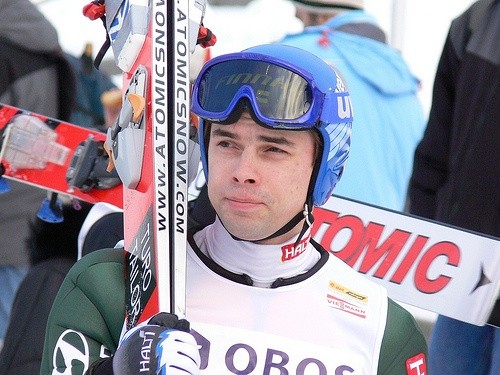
[190,53,324,131]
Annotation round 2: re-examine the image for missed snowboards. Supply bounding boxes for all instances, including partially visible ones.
[0,99,500,330]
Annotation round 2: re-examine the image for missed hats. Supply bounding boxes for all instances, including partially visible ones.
[292,0,364,14]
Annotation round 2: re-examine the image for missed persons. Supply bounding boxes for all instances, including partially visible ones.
[39,42,428,375]
[0,0,123,375]
[276,0,429,213]
[408,1,500,375]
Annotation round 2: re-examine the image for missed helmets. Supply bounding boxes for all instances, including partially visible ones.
[198,43,352,207]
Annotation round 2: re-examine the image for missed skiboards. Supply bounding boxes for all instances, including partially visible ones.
[82,0,217,322]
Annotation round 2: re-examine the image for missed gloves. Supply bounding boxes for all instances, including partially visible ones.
[113,312,201,375]
